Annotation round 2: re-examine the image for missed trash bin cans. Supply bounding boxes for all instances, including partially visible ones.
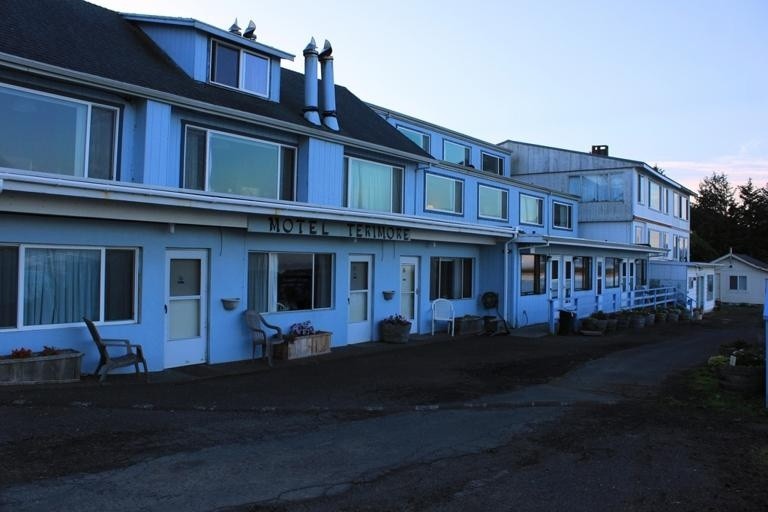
[558,310,576,336]
[694,307,702,321]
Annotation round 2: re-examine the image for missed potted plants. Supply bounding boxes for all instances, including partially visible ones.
[706,338,766,396]
[273,319,332,360]
[583,306,683,335]
[0,345,85,384]
[382,312,411,344]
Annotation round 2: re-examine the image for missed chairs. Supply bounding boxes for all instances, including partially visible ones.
[245,308,285,367]
[431,298,455,336]
[83,317,150,385]
